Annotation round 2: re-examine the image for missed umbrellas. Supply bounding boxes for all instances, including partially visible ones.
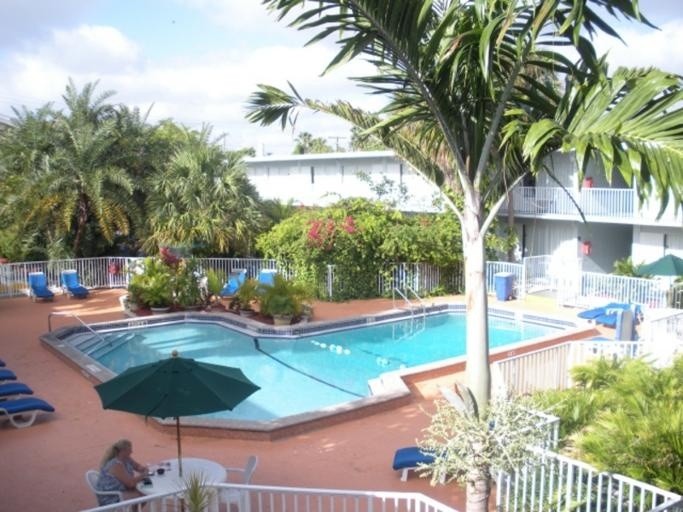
[93,348,262,512]
[632,254,683,276]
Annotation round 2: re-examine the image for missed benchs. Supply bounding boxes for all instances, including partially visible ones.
[575,302,642,328]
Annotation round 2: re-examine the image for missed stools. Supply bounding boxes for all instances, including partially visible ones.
[392,445,450,482]
[0,358,55,430]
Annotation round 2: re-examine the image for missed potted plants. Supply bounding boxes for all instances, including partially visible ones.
[119,243,316,326]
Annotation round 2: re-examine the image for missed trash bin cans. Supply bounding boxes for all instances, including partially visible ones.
[494,272,514,300]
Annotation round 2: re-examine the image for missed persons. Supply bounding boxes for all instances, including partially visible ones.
[91,439,151,512]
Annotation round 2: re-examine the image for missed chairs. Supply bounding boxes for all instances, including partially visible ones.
[28,269,90,302]
[85,454,259,511]
